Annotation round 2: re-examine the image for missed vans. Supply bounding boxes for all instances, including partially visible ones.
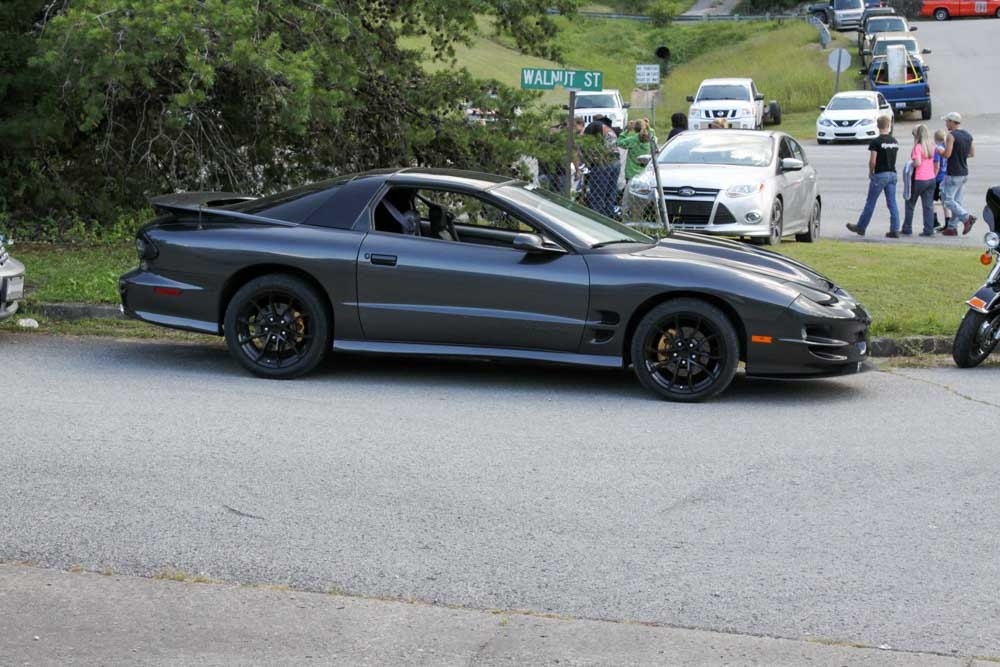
[857,7,896,40]
[825,0,870,31]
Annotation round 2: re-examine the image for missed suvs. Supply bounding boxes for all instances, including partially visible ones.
[808,0,883,25]
[562,90,629,135]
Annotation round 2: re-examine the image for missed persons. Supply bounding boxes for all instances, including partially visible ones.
[932,129,951,231]
[618,118,657,181]
[936,111,977,235]
[668,113,688,139]
[847,115,900,238]
[902,124,935,236]
[711,119,729,129]
[538,113,620,217]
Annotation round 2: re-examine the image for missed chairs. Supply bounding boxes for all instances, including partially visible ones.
[869,22,884,32]
[579,97,593,107]
[888,21,902,28]
[428,205,454,241]
[403,209,421,237]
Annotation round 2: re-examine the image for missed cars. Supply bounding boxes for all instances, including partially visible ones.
[0,233,27,322]
[854,16,919,54]
[860,35,932,66]
[815,90,896,144]
[685,77,765,130]
[621,127,821,247]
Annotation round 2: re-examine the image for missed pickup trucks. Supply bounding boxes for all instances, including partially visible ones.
[918,0,1000,21]
[859,55,932,121]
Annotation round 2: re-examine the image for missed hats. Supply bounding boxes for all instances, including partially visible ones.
[939,112,961,123]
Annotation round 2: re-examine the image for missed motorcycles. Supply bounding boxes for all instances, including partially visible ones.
[952,185,1000,369]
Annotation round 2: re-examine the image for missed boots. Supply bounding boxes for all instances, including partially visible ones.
[937,216,951,231]
[932,213,941,228]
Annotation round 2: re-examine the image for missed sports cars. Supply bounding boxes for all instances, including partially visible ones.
[118,165,873,403]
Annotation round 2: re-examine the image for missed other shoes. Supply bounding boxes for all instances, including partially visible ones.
[962,215,977,234]
[942,228,958,236]
[846,223,866,236]
[919,231,935,236]
[886,231,899,238]
[899,230,908,235]
